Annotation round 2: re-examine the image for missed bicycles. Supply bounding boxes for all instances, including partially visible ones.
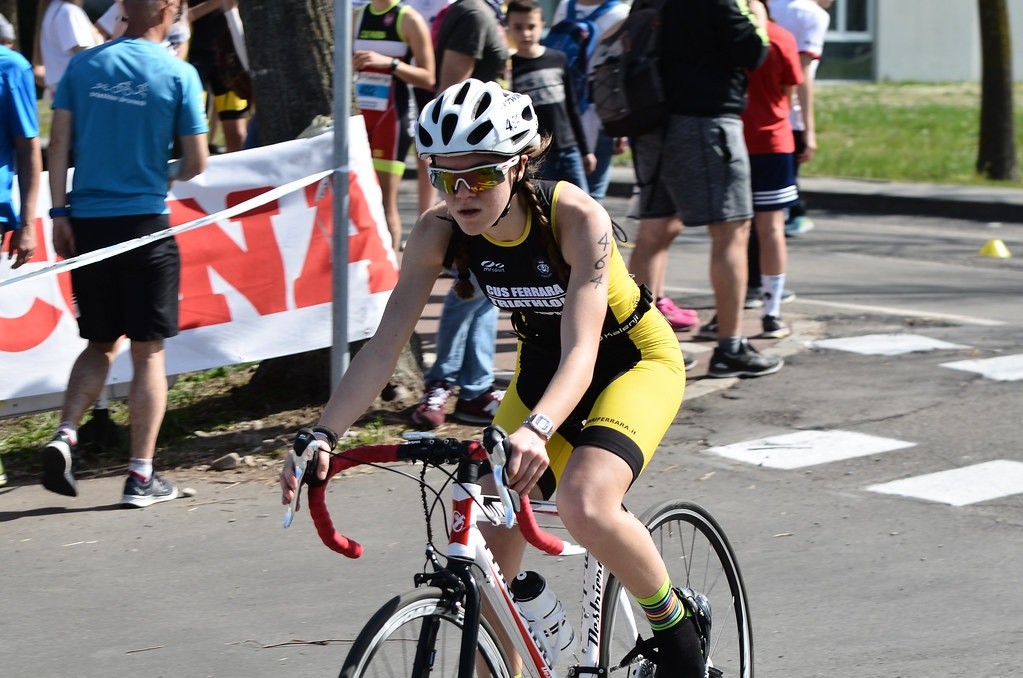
[281,418,752,678]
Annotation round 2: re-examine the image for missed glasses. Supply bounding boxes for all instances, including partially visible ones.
[427,155,522,197]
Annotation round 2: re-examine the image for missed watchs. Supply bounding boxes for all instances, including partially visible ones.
[388,58,400,74]
[522,414,554,442]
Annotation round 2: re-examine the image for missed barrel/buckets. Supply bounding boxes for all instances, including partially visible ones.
[353,67,393,112]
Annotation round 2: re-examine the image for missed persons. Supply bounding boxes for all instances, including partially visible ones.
[0,46,43,484]
[279,79,712,678]
[0,0,257,170]
[43,0,209,508]
[353,0,835,432]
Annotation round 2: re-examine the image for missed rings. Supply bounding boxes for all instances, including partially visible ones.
[25,256,32,261]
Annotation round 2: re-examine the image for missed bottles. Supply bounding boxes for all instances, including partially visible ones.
[510,570,577,664]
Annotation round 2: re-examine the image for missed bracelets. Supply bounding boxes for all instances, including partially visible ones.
[115,16,129,23]
[49,207,71,218]
[308,425,338,451]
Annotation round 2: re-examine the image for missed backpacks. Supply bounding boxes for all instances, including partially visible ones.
[544,0,626,116]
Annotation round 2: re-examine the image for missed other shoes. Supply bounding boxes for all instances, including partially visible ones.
[745,286,795,308]
[762,313,790,338]
[699,312,719,340]
[784,215,813,237]
[656,296,701,332]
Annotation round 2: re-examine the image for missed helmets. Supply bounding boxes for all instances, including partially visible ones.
[415,76,542,161]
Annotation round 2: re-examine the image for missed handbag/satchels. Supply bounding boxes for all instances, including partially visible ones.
[592,53,645,138]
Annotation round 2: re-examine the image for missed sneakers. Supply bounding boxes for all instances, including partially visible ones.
[707,336,784,378]
[682,351,699,371]
[655,585,713,678]
[121,469,178,508]
[39,432,79,497]
[455,383,507,424]
[412,379,454,430]
